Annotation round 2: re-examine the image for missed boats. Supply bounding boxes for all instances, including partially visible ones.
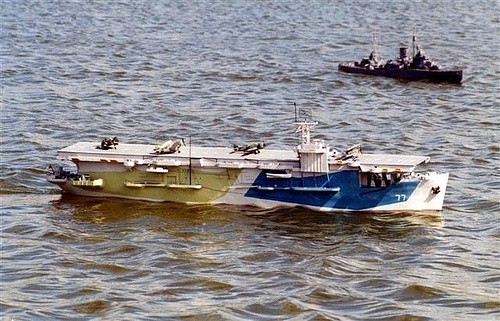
[47,101,451,215]
[339,30,463,83]
